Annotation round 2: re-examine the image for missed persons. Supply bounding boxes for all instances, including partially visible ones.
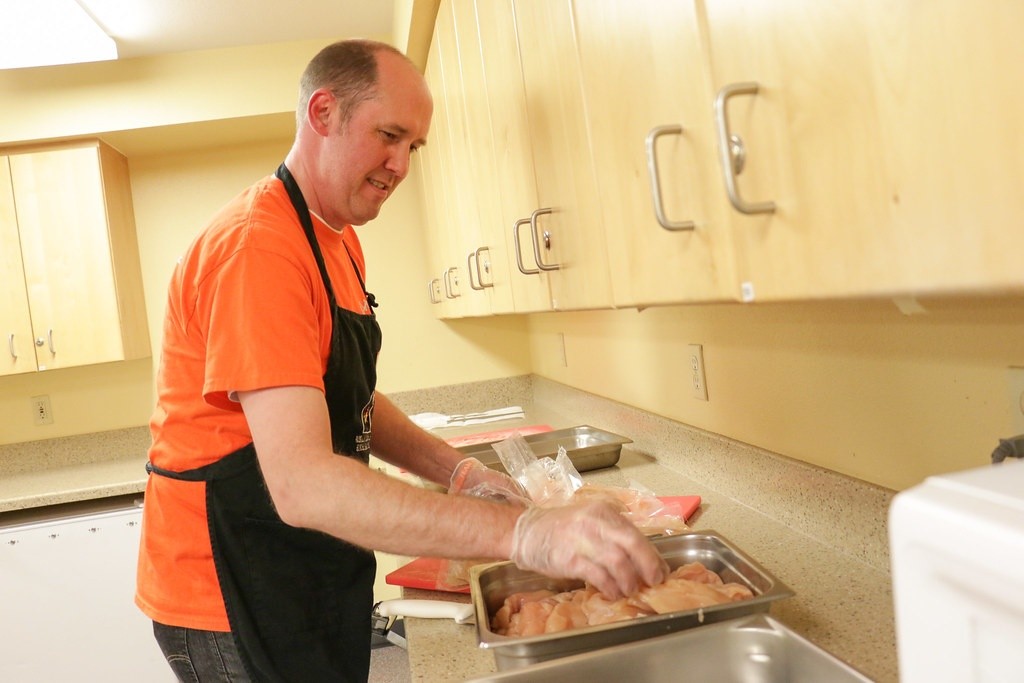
[135,40,669,683]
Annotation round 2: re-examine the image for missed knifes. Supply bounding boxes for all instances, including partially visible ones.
[375,598,476,631]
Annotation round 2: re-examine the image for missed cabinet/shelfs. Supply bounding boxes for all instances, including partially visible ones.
[0,140,153,378]
[0,498,178,683]
[422,0,1024,318]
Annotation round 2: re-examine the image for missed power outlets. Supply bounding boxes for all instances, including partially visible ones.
[687,343,708,401]
